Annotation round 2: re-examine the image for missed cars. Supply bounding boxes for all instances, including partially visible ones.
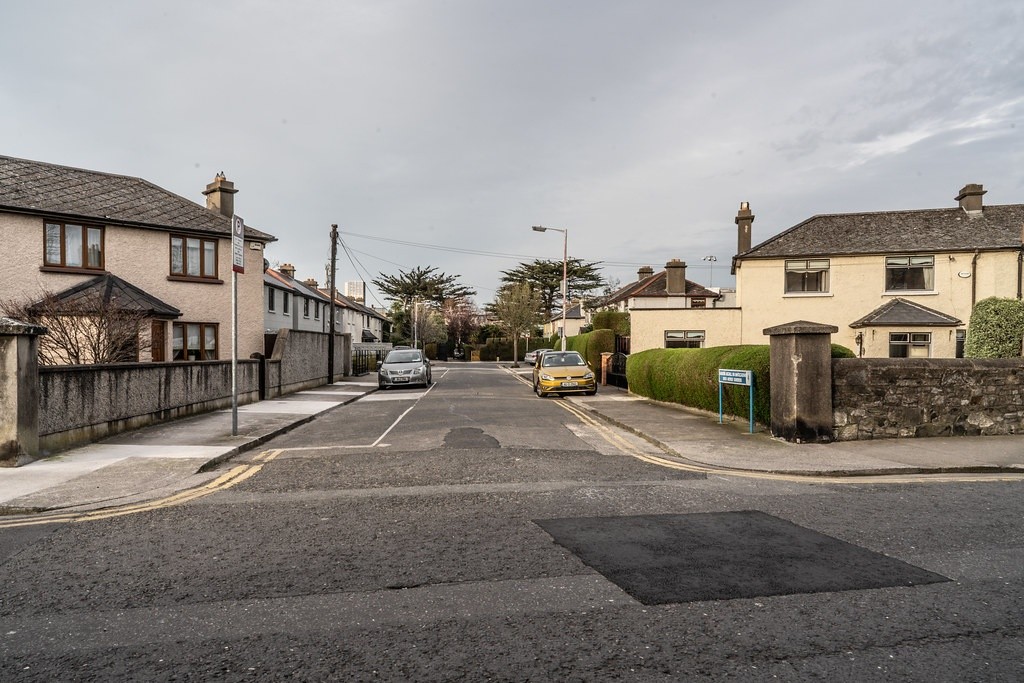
[377,348,432,389]
[453,349,465,359]
[524,348,553,366]
[531,350,597,398]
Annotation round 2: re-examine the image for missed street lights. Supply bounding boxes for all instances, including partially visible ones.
[702,255,718,287]
[531,225,570,351]
[413,297,432,349]
